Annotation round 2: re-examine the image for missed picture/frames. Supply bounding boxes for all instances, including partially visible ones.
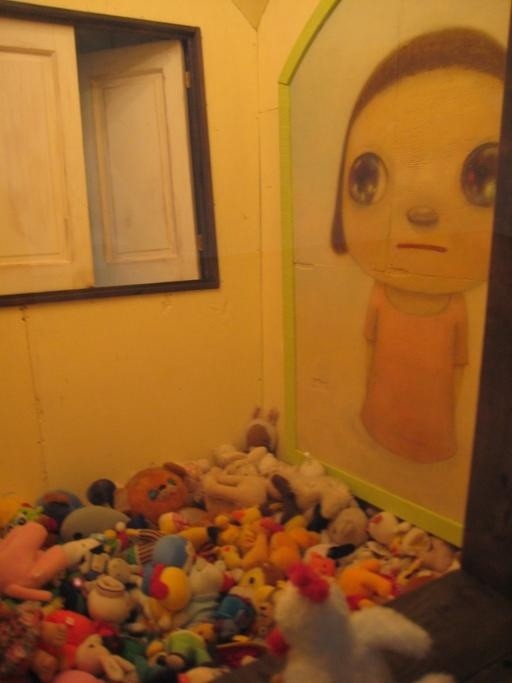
[271,1,510,550]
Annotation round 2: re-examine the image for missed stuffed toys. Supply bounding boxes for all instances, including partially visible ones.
[0,404,466,683]
[2,404,466,683]
